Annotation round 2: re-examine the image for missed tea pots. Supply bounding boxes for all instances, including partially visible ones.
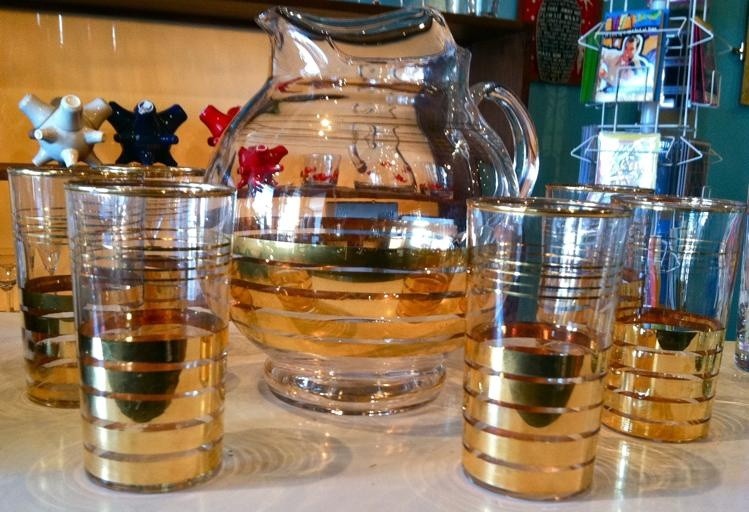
[202,5,541,414]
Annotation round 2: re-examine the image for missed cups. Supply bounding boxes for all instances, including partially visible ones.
[6,162,241,496]
[459,183,748,502]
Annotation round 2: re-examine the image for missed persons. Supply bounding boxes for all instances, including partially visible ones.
[602,35,648,93]
